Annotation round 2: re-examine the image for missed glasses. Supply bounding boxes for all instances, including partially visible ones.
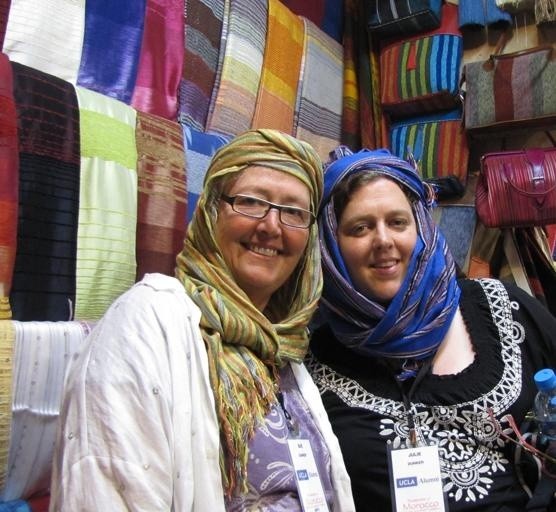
[220,192,318,230]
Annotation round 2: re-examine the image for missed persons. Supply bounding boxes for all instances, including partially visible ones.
[304,146,556,512]
[47,128,357,512]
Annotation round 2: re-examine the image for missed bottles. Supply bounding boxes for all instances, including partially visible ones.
[532,367,555,440]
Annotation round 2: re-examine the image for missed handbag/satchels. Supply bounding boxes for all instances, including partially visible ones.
[475,147,556,230]
[377,3,463,118]
[420,204,477,278]
[459,27,556,135]
[386,112,470,200]
[362,0,444,40]
